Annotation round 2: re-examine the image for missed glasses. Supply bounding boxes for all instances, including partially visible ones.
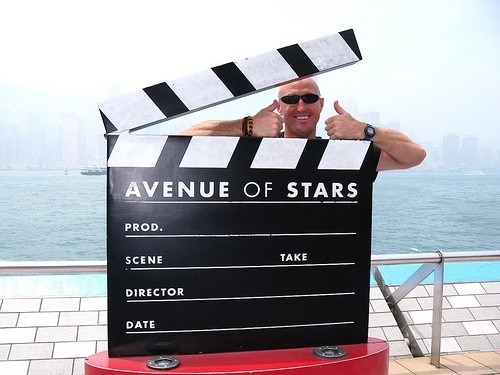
[278,93,320,104]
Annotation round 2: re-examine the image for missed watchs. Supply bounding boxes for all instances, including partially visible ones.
[361,123,376,140]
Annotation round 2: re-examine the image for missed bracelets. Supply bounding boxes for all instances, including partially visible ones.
[241,115,253,137]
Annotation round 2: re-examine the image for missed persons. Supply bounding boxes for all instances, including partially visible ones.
[177,77,427,182]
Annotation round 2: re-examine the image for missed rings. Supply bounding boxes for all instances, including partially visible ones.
[280,124,283,130]
